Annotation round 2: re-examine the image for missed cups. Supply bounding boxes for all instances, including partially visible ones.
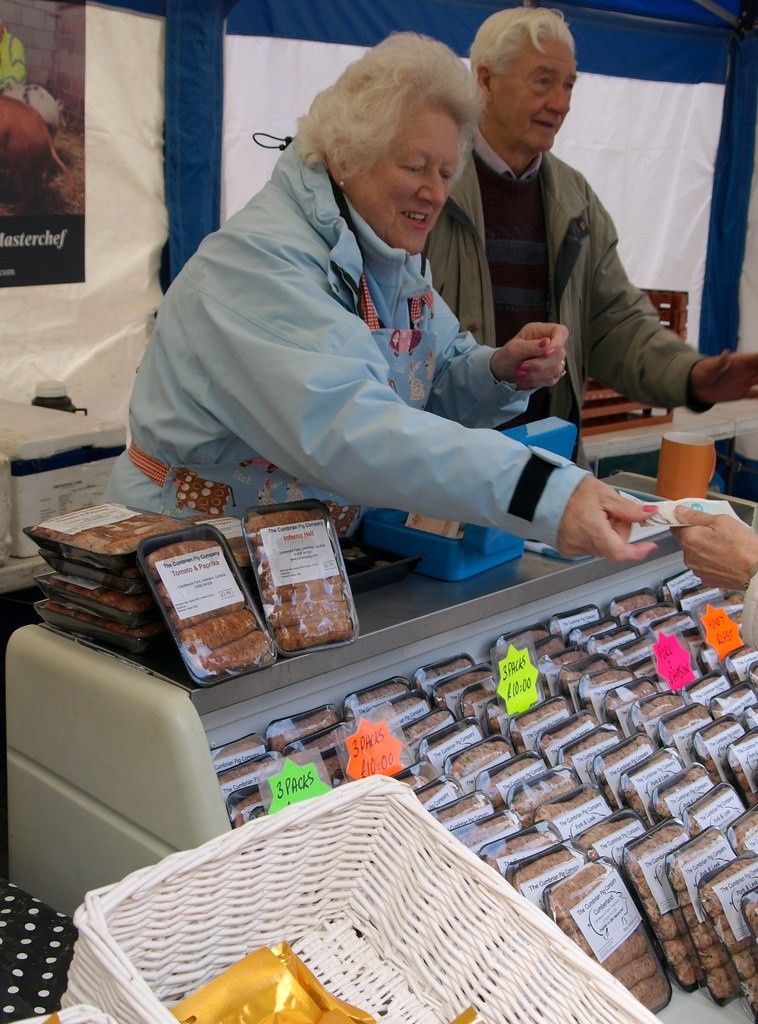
[655,433,717,502]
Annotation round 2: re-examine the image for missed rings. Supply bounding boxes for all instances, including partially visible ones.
[561,360,566,376]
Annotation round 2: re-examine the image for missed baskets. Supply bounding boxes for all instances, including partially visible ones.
[60,774,660,1024]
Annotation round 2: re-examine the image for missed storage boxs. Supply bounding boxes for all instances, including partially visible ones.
[0,399,129,558]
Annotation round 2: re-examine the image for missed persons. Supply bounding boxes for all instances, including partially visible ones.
[421,7,758,478]
[670,505,758,648]
[0,20,26,90]
[98,31,658,562]
